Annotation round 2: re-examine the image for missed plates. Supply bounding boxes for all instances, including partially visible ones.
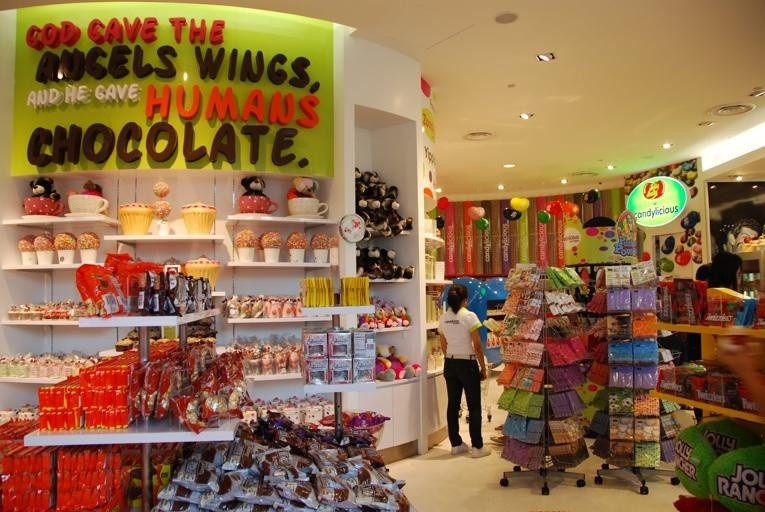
[64,213,105,217]
[21,215,58,219]
[236,212,273,216]
[286,214,325,219]
[287,198,328,215]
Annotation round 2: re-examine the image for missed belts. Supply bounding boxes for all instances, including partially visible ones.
[447,355,476,362]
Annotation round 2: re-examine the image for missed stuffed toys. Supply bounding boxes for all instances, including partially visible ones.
[287,177,319,200]
[29,176,60,201]
[240,176,266,197]
[63,179,103,196]
[374,345,422,382]
[355,168,415,279]
[357,297,412,328]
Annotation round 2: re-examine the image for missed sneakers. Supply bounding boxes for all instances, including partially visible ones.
[452,442,471,455]
[471,446,493,458]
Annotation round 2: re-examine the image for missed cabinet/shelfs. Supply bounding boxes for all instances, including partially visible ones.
[649,322,765,425]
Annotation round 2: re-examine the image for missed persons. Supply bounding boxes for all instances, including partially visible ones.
[436,283,492,459]
[716,337,765,412]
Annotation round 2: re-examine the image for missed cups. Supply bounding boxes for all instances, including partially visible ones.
[56,249,75,264]
[24,196,65,215]
[67,194,109,213]
[79,248,97,264]
[264,247,280,262]
[21,251,37,265]
[312,248,329,263]
[237,195,279,214]
[35,251,54,264]
[289,248,305,262]
[238,247,254,262]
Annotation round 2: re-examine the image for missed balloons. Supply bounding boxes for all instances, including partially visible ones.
[468,189,602,231]
